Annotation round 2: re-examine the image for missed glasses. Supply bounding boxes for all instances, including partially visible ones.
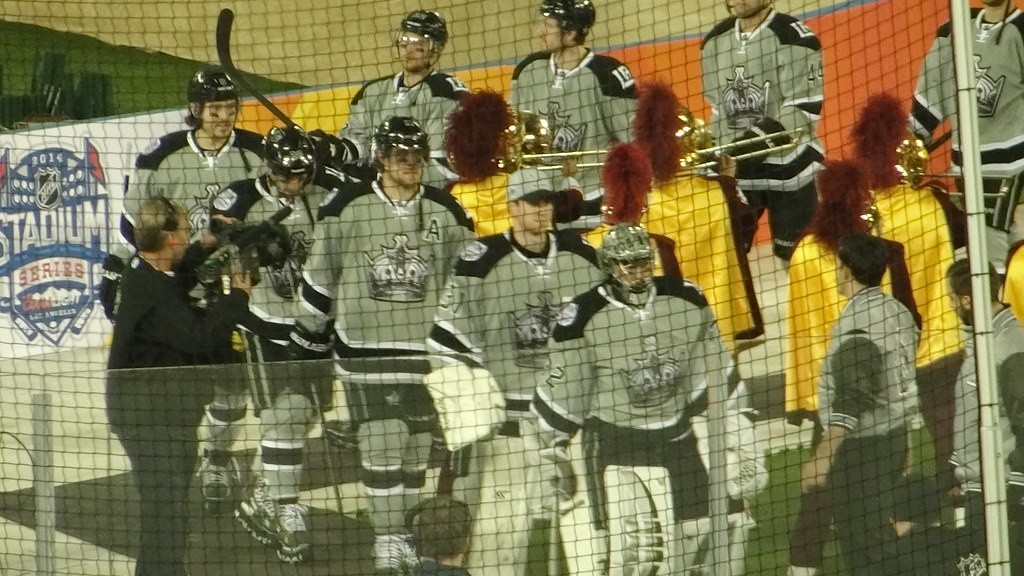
[153,194,176,219]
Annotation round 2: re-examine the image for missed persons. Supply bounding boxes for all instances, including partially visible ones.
[103,192,252,576]
[786,235,927,576]
[118,0,969,576]
[526,224,767,575]
[699,0,824,274]
[939,257,1024,576]
[907,0,1024,295]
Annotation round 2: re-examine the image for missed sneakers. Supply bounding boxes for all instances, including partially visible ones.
[198,448,240,515]
[238,483,311,564]
[371,531,425,576]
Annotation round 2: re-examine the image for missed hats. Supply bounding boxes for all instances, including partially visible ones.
[505,170,554,201]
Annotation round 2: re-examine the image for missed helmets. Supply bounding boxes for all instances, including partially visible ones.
[597,224,657,274]
[401,9,448,43]
[541,0,596,36]
[266,127,316,179]
[376,116,428,157]
[187,70,239,104]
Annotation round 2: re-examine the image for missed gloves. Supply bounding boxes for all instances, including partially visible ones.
[688,411,769,499]
[518,417,578,521]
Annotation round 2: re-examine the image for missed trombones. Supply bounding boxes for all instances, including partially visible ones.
[517,145,611,170]
[900,168,1011,202]
[684,126,808,174]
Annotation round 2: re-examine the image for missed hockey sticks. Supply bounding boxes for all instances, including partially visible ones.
[310,380,353,550]
[215,5,299,128]
